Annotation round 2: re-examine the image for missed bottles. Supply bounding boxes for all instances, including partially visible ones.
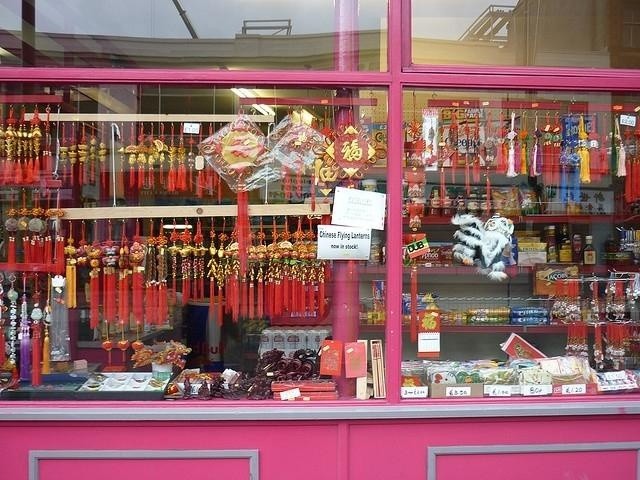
[543,224,597,265]
[606,238,615,252]
[426,186,494,216]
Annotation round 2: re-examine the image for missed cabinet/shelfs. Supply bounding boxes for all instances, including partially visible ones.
[0,200,640,399]
[1,424,342,480]
[342,412,640,480]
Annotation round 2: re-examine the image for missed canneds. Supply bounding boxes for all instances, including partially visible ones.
[479,194,494,218]
[468,193,479,217]
